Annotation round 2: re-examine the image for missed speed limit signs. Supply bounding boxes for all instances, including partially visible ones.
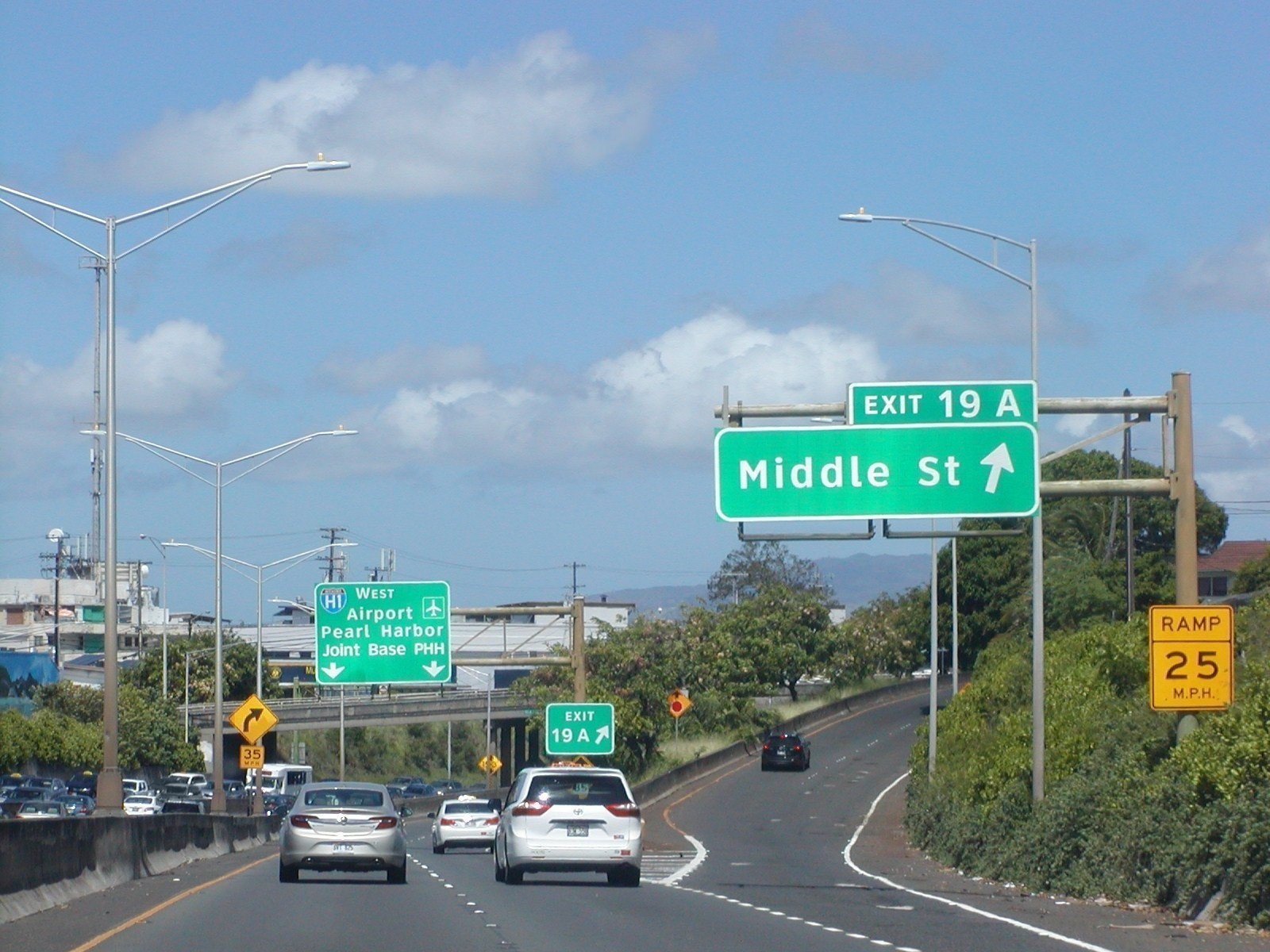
[1147,603,1236,713]
[239,744,265,770]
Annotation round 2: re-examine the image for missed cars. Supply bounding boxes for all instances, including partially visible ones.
[427,794,502,855]
[384,775,484,798]
[274,781,408,885]
[0,773,298,818]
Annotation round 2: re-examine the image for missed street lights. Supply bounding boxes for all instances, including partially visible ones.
[139,533,169,701]
[160,541,360,815]
[839,205,1046,812]
[0,154,352,812]
[80,425,360,819]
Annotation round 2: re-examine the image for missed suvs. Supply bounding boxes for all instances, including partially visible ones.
[761,729,810,772]
[486,764,645,887]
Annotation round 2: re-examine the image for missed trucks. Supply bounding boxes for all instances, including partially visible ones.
[243,763,313,796]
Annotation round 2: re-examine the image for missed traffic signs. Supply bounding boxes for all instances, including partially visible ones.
[313,579,453,685]
[712,422,1043,519]
[545,702,617,756]
